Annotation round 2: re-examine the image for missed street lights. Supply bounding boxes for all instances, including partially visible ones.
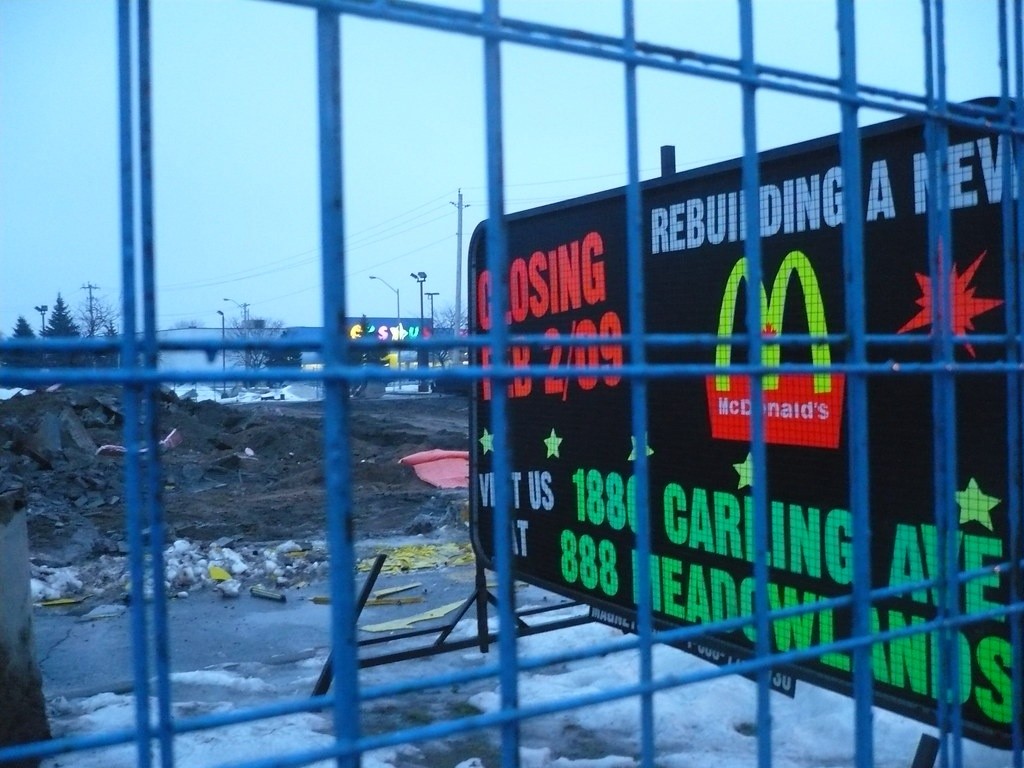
[223,298,247,330]
[411,271,426,336]
[217,311,226,394]
[371,276,402,389]
